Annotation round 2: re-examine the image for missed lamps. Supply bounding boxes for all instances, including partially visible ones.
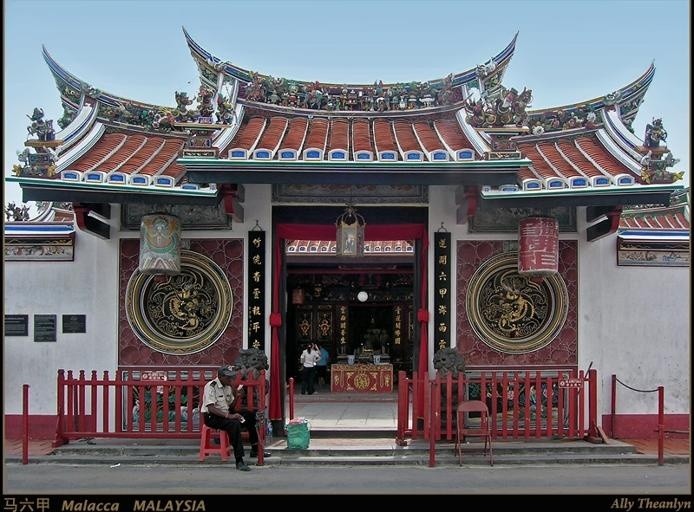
[138,203,182,275]
[335,184,366,259]
[518,214,559,276]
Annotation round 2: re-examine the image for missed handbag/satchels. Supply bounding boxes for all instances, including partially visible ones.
[286,420,310,449]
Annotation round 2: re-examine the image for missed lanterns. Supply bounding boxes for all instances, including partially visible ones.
[516,213,560,284]
[138,210,184,282]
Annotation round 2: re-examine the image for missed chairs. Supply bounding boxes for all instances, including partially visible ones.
[453,400,494,467]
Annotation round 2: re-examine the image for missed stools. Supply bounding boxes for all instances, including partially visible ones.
[199,424,232,462]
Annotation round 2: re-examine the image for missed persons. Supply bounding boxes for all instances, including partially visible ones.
[201,365,271,471]
[8,68,686,184]
[300,341,330,395]
[2,200,31,222]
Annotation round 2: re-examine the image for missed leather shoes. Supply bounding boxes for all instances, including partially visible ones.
[236,462,251,471]
[250,451,271,457]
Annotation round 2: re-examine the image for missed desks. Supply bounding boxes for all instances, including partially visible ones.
[330,363,394,393]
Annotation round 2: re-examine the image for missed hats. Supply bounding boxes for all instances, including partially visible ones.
[218,367,236,377]
